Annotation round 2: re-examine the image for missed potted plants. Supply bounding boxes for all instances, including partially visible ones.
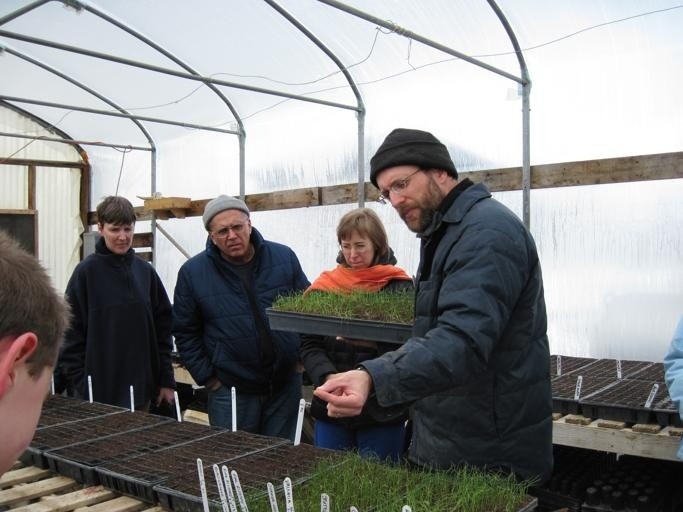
[215,446,543,512]
[265,284,414,345]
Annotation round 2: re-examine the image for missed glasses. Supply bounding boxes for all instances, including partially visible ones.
[376,166,426,206]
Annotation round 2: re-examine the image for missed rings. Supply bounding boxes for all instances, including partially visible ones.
[335,412,341,418]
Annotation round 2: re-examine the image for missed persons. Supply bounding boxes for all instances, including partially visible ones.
[302,205,416,464]
[171,192,314,444]
[50,194,176,418]
[0,228,76,482]
[311,127,555,505]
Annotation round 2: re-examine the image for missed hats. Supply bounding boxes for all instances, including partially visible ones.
[369,129,458,190]
[202,194,250,232]
